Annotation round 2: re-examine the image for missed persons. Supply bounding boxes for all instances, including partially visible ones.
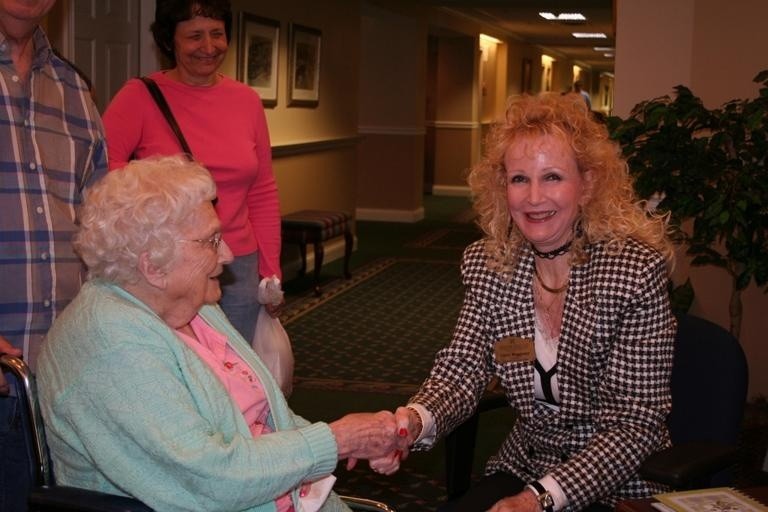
[345,92,696,512]
[100,0,282,347]
[35,154,413,512]
[570,79,592,112]
[1,0,106,510]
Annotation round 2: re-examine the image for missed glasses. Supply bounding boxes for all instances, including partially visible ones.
[174,228,224,254]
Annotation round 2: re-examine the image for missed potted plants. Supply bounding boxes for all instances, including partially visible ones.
[593,70,768,490]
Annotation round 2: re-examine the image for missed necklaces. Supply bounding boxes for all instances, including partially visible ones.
[531,256,571,293]
[529,231,584,259]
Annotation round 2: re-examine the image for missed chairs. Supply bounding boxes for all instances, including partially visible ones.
[446,310,750,512]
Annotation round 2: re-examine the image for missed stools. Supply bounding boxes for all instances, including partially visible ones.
[281,209,354,295]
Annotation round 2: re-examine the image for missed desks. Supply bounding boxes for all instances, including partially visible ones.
[616,489,768,512]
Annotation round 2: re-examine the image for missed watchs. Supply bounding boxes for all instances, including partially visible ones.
[528,480,555,512]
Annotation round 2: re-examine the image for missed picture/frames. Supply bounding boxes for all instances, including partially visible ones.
[286,20,322,108]
[237,11,280,106]
[522,58,532,93]
